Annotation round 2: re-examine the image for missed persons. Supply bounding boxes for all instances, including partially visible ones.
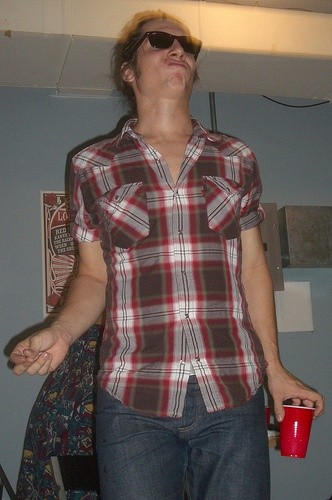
[9,9,325,500]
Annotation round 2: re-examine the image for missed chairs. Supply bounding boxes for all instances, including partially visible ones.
[11,325,106,500]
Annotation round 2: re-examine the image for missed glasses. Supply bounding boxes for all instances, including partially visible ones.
[128,31,202,64]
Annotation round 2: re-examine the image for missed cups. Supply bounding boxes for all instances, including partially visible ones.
[265,406,272,427]
[280,404,316,458]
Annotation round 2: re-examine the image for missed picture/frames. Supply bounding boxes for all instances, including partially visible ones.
[40,190,106,318]
